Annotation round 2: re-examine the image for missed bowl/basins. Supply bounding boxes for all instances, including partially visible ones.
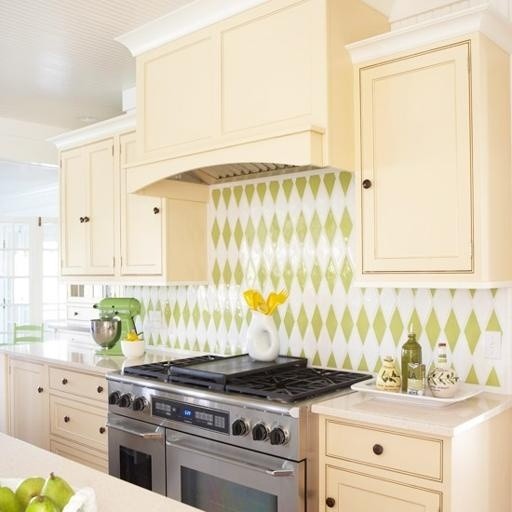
[121,341,146,357]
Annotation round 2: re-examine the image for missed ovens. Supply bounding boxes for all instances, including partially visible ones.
[108,380,317,512]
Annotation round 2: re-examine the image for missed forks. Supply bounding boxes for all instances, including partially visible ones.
[267,288,290,314]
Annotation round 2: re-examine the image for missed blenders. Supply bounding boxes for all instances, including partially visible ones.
[90,296,141,356]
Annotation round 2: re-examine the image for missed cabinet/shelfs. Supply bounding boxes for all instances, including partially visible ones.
[117,128,207,285]
[319,407,512,511]
[1,344,108,474]
[58,134,117,284]
[351,33,511,288]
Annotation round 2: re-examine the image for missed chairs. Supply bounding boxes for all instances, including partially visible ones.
[12,322,44,346]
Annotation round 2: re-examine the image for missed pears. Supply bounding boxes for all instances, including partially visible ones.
[0,472,74,512]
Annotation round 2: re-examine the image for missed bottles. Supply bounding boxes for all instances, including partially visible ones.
[376,323,459,398]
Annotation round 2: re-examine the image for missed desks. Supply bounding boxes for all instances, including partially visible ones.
[0,433,210,512]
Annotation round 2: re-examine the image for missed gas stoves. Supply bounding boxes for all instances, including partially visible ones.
[106,354,372,404]
